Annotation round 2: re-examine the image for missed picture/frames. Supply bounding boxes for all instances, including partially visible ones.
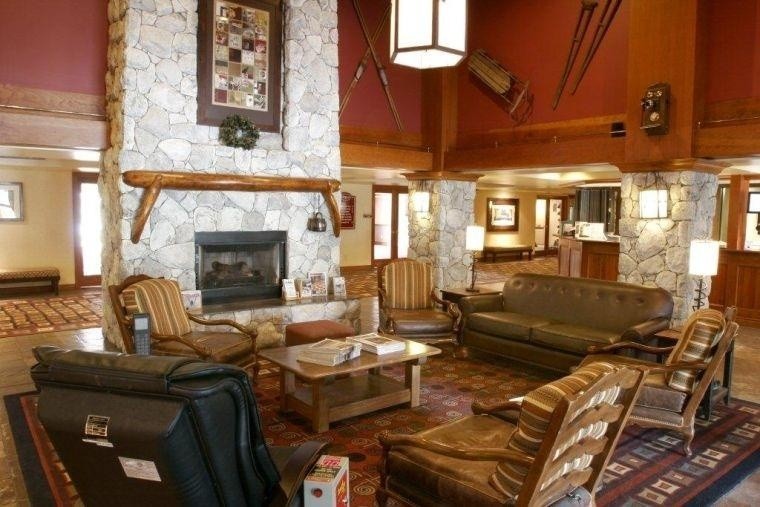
[0,182,24,222]
[486,198,519,232]
[339,192,357,229]
[197,0,282,133]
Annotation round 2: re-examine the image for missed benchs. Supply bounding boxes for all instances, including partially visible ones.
[484,246,533,262]
[0,267,61,295]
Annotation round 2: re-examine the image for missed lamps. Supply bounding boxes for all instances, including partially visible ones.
[639,189,668,219]
[388,0,470,71]
[414,179,430,212]
[466,224,485,292]
[688,240,719,311]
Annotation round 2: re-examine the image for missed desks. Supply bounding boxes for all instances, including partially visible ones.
[654,326,734,422]
[439,285,503,312]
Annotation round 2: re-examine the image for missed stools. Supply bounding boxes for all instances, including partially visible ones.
[286,320,357,346]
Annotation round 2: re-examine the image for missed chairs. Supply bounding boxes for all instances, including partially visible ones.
[375,360,649,507]
[30,346,330,506]
[377,257,461,359]
[109,274,259,385]
[571,305,740,458]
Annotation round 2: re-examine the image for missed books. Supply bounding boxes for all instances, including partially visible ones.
[297,338,361,366]
[346,332,406,356]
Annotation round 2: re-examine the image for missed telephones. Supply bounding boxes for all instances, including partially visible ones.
[133,312,151,355]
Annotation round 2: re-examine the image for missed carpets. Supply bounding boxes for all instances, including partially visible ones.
[2,341,760,506]
[0,295,103,338]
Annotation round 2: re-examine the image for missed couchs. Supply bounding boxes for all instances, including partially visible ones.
[458,272,674,374]
